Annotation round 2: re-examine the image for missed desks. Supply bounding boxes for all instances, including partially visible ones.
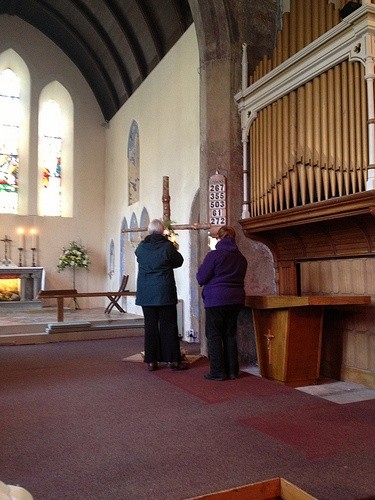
[242,294,370,385]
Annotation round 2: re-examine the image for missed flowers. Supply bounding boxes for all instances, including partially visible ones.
[162,220,176,236]
[58,241,89,271]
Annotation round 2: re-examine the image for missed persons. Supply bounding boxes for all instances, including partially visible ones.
[134,219,188,372]
[196,225,239,381]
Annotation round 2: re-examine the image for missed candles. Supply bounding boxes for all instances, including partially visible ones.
[18,229,24,248]
[32,229,37,248]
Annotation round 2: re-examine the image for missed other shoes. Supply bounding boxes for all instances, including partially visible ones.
[168,362,189,370]
[204,373,224,381]
[227,373,236,380]
[148,362,161,371]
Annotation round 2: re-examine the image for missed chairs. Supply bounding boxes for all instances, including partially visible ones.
[102,274,129,314]
